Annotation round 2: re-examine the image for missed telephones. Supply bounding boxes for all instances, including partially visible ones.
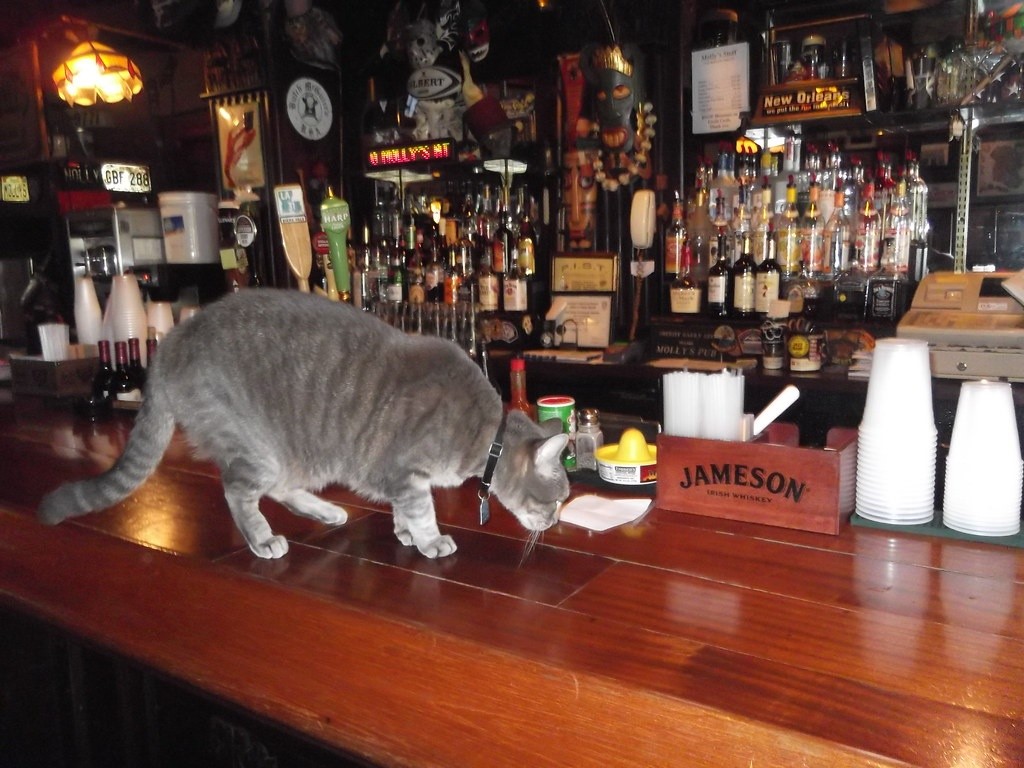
[630,189,656,250]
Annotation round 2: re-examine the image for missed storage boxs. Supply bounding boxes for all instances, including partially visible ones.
[8,345,99,400]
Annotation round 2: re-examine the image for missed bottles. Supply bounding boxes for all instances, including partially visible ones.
[575,407,605,471]
[370,300,502,401]
[703,7,1023,110]
[364,76,386,133]
[92,337,159,402]
[664,130,930,326]
[505,358,536,424]
[354,180,543,312]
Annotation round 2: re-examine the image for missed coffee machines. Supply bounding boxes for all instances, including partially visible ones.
[64,208,171,311]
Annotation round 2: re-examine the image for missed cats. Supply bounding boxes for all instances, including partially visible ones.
[38,285,574,558]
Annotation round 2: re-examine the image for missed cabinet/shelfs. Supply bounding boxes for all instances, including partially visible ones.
[352,0,1024,446]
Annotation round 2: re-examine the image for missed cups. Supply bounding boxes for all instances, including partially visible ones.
[854,337,938,526]
[178,305,201,322]
[37,324,71,362]
[537,395,576,471]
[942,381,1023,537]
[788,331,827,371]
[759,325,787,370]
[73,276,103,345]
[100,275,175,370]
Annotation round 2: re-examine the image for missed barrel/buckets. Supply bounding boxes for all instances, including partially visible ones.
[157,192,221,265]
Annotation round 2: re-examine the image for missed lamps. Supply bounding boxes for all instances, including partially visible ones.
[50,20,144,108]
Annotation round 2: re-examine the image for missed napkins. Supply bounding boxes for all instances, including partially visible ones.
[562,492,651,531]
